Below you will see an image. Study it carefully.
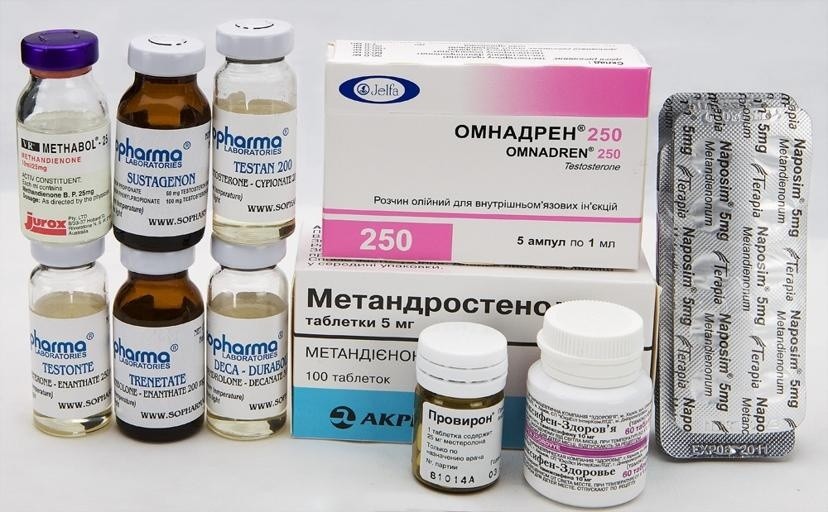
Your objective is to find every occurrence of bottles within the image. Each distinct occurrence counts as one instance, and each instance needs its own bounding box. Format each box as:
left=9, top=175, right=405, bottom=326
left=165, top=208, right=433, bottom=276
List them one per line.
left=205, top=233, right=288, bottom=441
left=113, top=243, right=205, bottom=443
left=16, top=28, right=111, bottom=245
left=522, top=298, right=653, bottom=508
left=212, top=18, right=299, bottom=246
left=28, top=237, right=112, bottom=438
left=410, top=321, right=509, bottom=494
left=112, top=31, right=211, bottom=253
